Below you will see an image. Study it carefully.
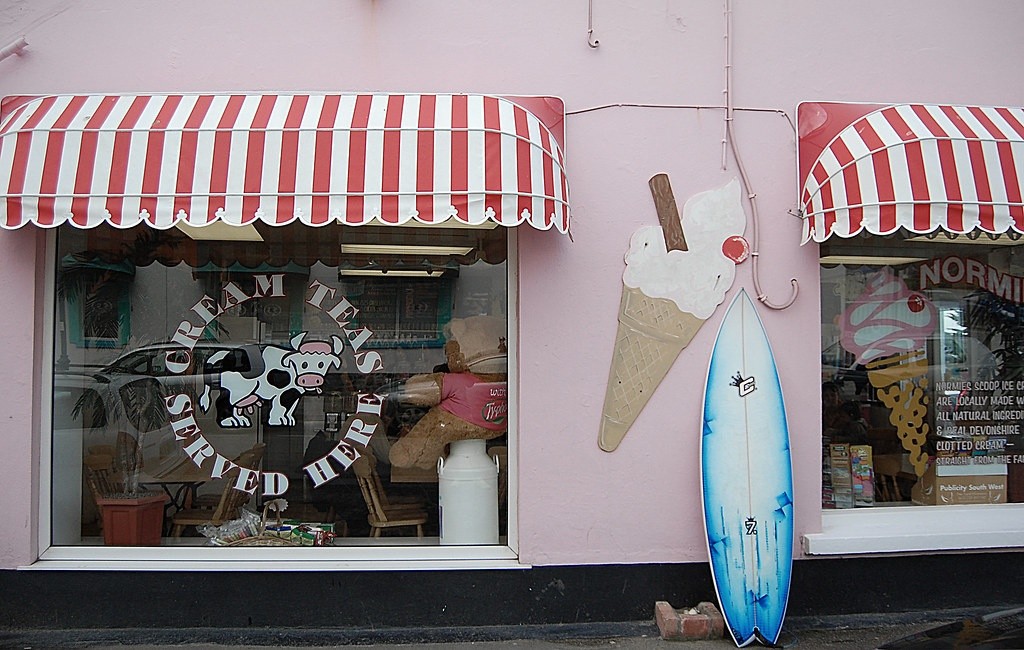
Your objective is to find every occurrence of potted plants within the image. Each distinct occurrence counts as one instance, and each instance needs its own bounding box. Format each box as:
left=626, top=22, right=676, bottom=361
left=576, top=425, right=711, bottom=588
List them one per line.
left=57, top=219, right=226, bottom=557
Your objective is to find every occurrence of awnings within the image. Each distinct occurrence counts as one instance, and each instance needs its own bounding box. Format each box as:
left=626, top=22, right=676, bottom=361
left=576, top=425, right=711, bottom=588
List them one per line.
left=0, top=93, right=574, bottom=238
left=794, top=101, right=1024, bottom=246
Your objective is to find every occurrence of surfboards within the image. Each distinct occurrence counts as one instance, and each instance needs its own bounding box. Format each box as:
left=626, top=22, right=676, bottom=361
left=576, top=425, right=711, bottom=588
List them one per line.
left=698, top=288, right=796, bottom=649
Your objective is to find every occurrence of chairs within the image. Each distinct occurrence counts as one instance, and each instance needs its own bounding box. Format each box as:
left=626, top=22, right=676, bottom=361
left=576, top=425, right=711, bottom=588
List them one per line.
left=85, top=452, right=132, bottom=528
left=348, top=445, right=432, bottom=539
left=169, top=443, right=267, bottom=537
left=868, top=451, right=903, bottom=503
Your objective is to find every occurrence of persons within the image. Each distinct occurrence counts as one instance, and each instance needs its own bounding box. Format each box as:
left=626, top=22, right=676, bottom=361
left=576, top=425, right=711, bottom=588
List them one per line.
left=822, top=362, right=969, bottom=460
left=305, top=393, right=416, bottom=540
left=160, top=345, right=249, bottom=372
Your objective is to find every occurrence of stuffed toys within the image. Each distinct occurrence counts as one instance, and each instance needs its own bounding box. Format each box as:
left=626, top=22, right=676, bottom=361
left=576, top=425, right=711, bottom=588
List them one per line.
left=390, top=314, right=508, bottom=470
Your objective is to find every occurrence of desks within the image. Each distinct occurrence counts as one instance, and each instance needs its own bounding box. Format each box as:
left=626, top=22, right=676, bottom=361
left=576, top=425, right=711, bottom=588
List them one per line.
left=388, top=459, right=439, bottom=492
left=126, top=448, right=234, bottom=539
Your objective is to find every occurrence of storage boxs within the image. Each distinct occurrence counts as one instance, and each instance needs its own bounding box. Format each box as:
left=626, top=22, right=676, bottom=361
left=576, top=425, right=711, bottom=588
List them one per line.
left=908, top=458, right=1009, bottom=505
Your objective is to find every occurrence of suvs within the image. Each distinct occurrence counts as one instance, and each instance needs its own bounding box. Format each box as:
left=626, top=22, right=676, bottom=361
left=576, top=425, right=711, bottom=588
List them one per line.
left=50, top=340, right=291, bottom=533
